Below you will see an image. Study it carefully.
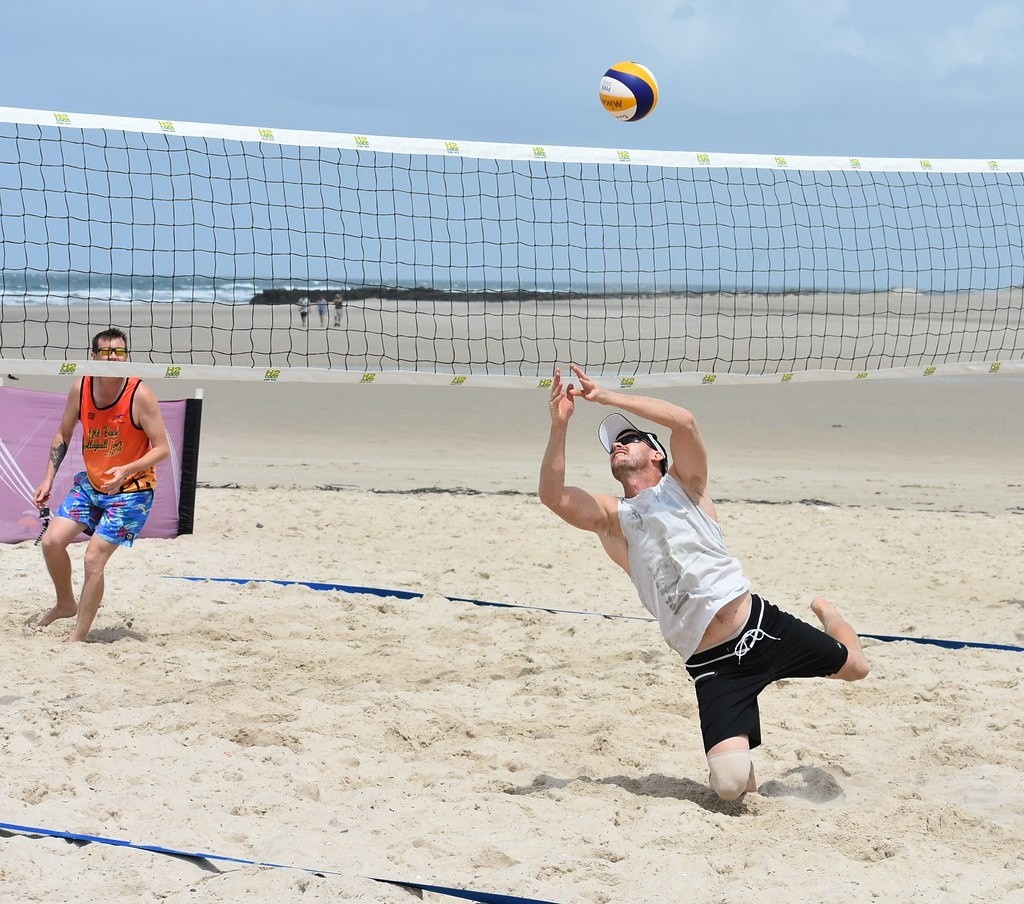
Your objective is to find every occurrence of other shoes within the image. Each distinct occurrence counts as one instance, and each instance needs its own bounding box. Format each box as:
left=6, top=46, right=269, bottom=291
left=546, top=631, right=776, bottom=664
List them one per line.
left=334, top=323, right=340, bottom=327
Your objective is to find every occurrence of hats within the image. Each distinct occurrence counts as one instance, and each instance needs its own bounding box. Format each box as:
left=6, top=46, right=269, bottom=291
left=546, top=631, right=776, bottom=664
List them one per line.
left=598, top=413, right=668, bottom=476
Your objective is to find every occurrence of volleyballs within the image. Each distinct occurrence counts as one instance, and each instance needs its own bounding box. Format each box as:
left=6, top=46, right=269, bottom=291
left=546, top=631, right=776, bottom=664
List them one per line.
left=598, top=60, right=659, bottom=123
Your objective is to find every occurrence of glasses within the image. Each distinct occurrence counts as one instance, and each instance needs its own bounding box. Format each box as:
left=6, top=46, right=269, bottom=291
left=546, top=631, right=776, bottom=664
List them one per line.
left=96, top=347, right=127, bottom=356
left=610, top=434, right=657, bottom=453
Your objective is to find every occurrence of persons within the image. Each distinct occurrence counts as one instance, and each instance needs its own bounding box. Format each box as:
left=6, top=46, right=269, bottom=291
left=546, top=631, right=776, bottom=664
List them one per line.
left=35, top=326, right=170, bottom=646
left=298, top=293, right=343, bottom=326
left=536, top=359, right=870, bottom=802
